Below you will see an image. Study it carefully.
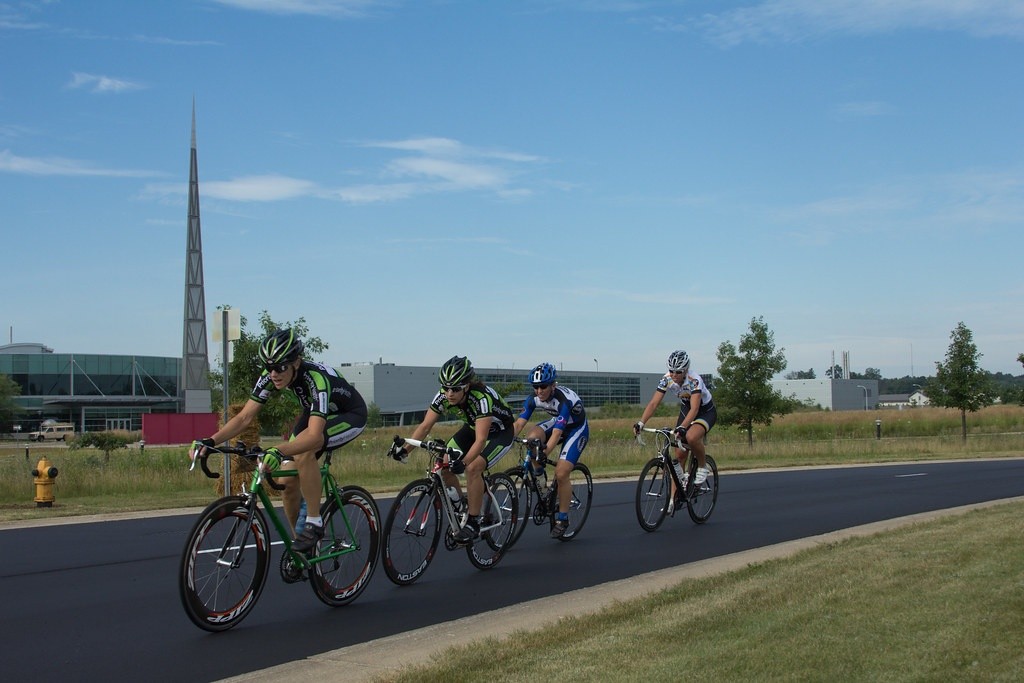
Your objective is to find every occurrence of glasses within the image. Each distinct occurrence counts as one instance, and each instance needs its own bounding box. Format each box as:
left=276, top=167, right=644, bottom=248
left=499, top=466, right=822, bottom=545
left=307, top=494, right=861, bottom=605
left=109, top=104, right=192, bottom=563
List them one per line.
left=532, top=383, right=552, bottom=389
left=441, top=385, right=467, bottom=392
left=265, top=362, right=292, bottom=373
left=669, top=369, right=685, bottom=374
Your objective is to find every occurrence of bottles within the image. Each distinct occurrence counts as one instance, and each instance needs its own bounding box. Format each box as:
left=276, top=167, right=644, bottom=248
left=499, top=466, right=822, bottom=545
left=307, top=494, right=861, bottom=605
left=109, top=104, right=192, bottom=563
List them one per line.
left=294, top=498, right=307, bottom=532
left=533, top=462, right=548, bottom=494
left=447, top=485, right=464, bottom=512
left=671, top=458, right=687, bottom=485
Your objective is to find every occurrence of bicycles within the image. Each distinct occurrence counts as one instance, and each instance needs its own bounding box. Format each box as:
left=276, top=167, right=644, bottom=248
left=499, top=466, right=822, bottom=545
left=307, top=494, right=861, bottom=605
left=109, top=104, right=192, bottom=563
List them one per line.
left=380, top=434, right=593, bottom=586
left=635, top=427, right=720, bottom=532
left=177, top=439, right=383, bottom=633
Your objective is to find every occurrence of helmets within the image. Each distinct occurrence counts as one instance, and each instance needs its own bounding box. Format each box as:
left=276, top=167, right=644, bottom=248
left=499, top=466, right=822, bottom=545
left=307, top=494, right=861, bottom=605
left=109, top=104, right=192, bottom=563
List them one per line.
left=667, top=350, right=691, bottom=372
left=437, top=355, right=476, bottom=386
left=258, top=328, right=304, bottom=365
left=527, top=362, right=557, bottom=385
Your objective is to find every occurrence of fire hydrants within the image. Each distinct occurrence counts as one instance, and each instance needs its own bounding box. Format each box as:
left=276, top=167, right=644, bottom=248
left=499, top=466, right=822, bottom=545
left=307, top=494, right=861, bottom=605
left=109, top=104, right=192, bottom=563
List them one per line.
left=31, top=456, right=58, bottom=508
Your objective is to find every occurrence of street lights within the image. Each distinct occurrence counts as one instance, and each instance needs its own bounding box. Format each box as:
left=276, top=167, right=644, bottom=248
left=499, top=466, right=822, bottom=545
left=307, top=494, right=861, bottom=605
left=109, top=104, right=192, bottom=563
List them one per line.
left=594, top=359, right=598, bottom=371
left=857, top=385, right=868, bottom=411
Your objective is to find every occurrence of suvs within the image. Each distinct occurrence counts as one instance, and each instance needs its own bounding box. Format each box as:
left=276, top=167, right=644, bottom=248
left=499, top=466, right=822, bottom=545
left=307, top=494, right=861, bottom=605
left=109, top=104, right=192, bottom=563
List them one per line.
left=28, top=426, right=75, bottom=442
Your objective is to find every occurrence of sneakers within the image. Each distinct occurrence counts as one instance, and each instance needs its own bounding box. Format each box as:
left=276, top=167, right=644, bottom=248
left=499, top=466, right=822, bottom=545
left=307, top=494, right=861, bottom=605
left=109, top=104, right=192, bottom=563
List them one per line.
left=291, top=523, right=324, bottom=551
left=531, top=471, right=547, bottom=493
left=453, top=523, right=480, bottom=543
left=550, top=517, right=570, bottom=539
left=661, top=500, right=673, bottom=513
left=694, top=467, right=710, bottom=484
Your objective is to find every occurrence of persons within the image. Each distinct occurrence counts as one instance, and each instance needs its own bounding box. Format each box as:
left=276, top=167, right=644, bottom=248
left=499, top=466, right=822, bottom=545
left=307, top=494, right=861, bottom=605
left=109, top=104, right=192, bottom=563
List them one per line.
left=187, top=328, right=368, bottom=570
left=633, top=350, right=717, bottom=514
left=392, top=355, right=515, bottom=543
left=514, top=362, right=589, bottom=539
